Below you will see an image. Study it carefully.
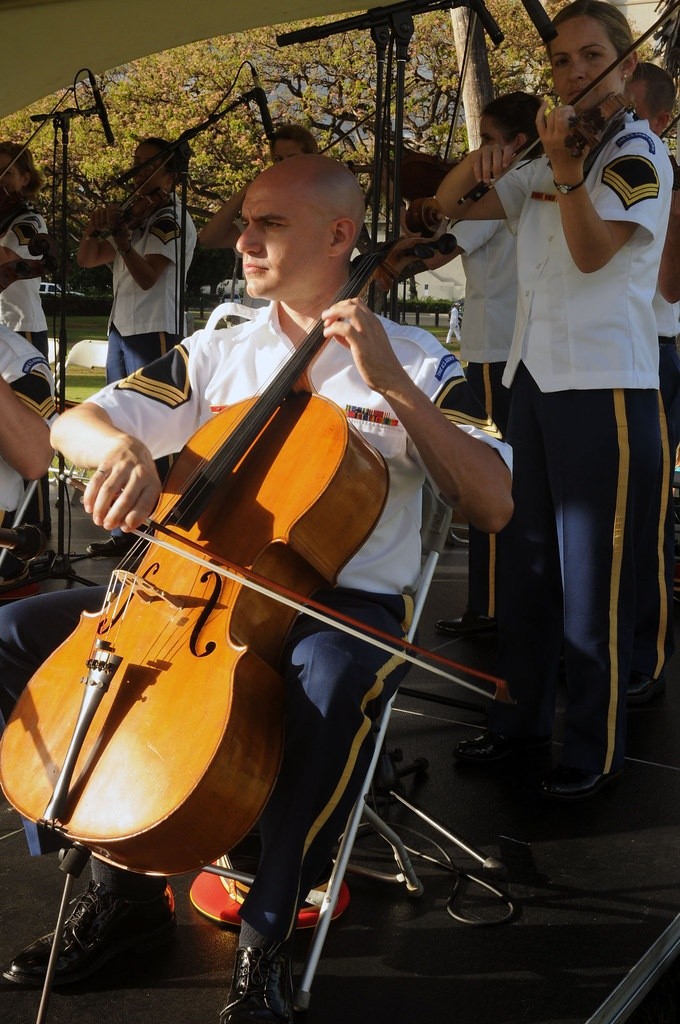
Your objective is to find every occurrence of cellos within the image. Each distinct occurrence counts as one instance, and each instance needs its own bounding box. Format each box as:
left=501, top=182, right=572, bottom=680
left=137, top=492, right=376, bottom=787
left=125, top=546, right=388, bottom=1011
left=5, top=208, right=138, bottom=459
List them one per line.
left=0, top=197, right=457, bottom=1024
left=0, top=233, right=60, bottom=294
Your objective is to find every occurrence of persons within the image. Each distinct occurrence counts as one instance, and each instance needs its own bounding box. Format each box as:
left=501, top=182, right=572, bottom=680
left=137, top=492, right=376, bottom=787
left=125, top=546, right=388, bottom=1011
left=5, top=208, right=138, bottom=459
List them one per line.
left=0, top=141, right=51, bottom=538
left=196, top=126, right=318, bottom=323
left=0, top=153, right=512, bottom=1024
left=380, top=0, right=680, bottom=793
left=0, top=324, right=59, bottom=556
left=446, top=303, right=463, bottom=344
left=77, top=137, right=196, bottom=556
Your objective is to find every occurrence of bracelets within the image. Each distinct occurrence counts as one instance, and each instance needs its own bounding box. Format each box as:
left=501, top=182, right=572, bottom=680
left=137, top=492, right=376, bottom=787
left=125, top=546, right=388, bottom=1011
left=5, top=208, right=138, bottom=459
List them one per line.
left=117, top=243, right=131, bottom=255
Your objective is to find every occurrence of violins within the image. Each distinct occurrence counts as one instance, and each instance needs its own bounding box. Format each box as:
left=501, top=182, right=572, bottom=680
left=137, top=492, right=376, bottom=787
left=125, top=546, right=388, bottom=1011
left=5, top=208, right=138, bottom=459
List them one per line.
left=126, top=184, right=171, bottom=230
left=546, top=91, right=630, bottom=171
left=0, top=191, right=27, bottom=234
left=348, top=152, right=459, bottom=200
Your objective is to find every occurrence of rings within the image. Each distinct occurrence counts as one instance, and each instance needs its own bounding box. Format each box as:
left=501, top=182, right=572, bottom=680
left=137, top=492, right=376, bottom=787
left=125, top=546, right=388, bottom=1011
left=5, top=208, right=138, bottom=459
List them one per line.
left=97, top=469, right=108, bottom=478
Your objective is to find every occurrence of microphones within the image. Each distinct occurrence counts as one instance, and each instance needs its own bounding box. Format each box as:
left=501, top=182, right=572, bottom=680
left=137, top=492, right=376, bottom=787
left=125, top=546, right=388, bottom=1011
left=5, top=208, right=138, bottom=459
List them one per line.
left=466, top=0, right=505, bottom=45
left=521, top=0, right=559, bottom=43
left=251, top=66, right=276, bottom=140
left=88, top=71, right=115, bottom=143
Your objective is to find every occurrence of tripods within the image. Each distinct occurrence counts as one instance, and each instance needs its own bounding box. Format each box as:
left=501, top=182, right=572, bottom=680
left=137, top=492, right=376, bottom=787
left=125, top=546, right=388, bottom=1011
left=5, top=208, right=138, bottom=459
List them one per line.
left=0, top=104, right=106, bottom=590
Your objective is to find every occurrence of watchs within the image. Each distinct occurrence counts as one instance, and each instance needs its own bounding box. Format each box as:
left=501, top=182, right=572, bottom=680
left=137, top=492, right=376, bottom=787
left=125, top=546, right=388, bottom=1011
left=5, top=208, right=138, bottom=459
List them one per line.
left=552, top=177, right=586, bottom=193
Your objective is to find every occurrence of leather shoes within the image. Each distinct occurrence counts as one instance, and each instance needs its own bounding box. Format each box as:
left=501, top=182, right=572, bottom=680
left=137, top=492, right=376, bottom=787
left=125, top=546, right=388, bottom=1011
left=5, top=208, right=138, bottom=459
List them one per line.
left=90, top=532, right=149, bottom=559
left=435, top=608, right=500, bottom=636
left=538, top=759, right=625, bottom=799
left=624, top=669, right=665, bottom=703
left=216, top=945, right=293, bottom=1024
left=452, top=720, right=552, bottom=761
left=4, top=878, right=176, bottom=986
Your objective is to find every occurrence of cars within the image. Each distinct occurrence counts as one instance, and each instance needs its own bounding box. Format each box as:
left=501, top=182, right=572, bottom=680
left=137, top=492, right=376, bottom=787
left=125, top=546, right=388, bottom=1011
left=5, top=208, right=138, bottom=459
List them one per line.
left=38, top=283, right=84, bottom=297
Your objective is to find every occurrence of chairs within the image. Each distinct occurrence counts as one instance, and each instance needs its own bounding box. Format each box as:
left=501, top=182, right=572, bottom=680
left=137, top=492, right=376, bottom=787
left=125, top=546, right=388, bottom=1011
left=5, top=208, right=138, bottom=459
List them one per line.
left=201, top=476, right=452, bottom=1011
left=0, top=338, right=109, bottom=565
